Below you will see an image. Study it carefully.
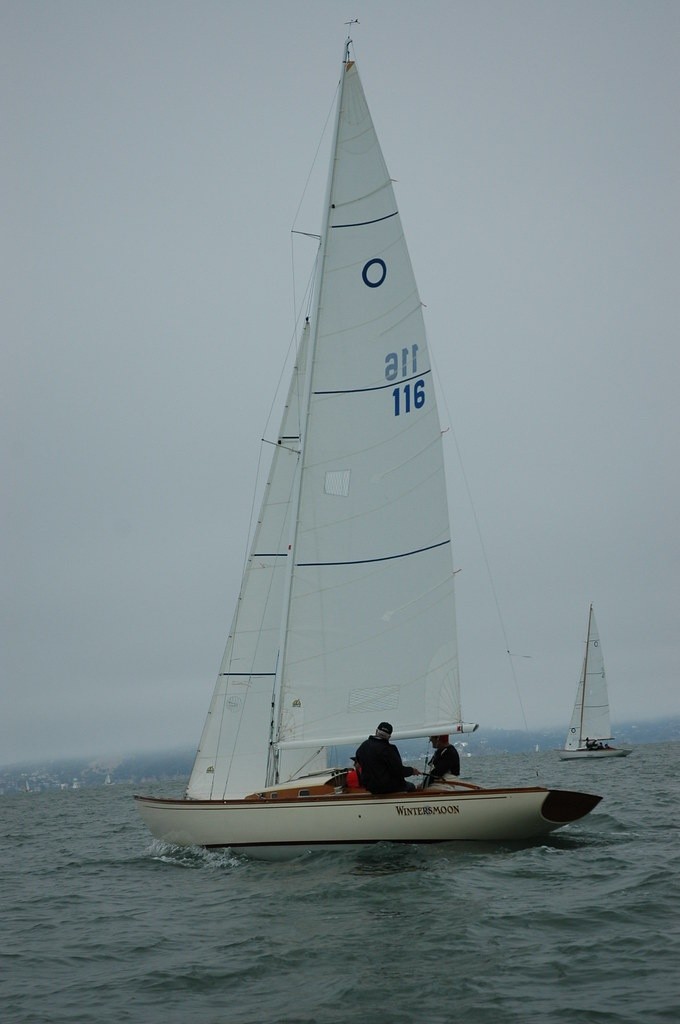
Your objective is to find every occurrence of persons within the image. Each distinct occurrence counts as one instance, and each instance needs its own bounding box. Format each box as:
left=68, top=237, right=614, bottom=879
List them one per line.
left=343, top=756, right=367, bottom=794
left=586, top=737, right=611, bottom=750
left=356, top=721, right=419, bottom=796
left=417, top=734, right=460, bottom=791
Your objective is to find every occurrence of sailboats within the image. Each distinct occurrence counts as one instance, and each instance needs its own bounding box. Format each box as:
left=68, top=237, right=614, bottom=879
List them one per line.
left=129, top=18, right=606, bottom=862
left=553, top=600, right=633, bottom=761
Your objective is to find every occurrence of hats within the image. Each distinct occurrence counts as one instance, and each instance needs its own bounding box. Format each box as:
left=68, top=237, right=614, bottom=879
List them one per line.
left=378, top=722, right=393, bottom=734
